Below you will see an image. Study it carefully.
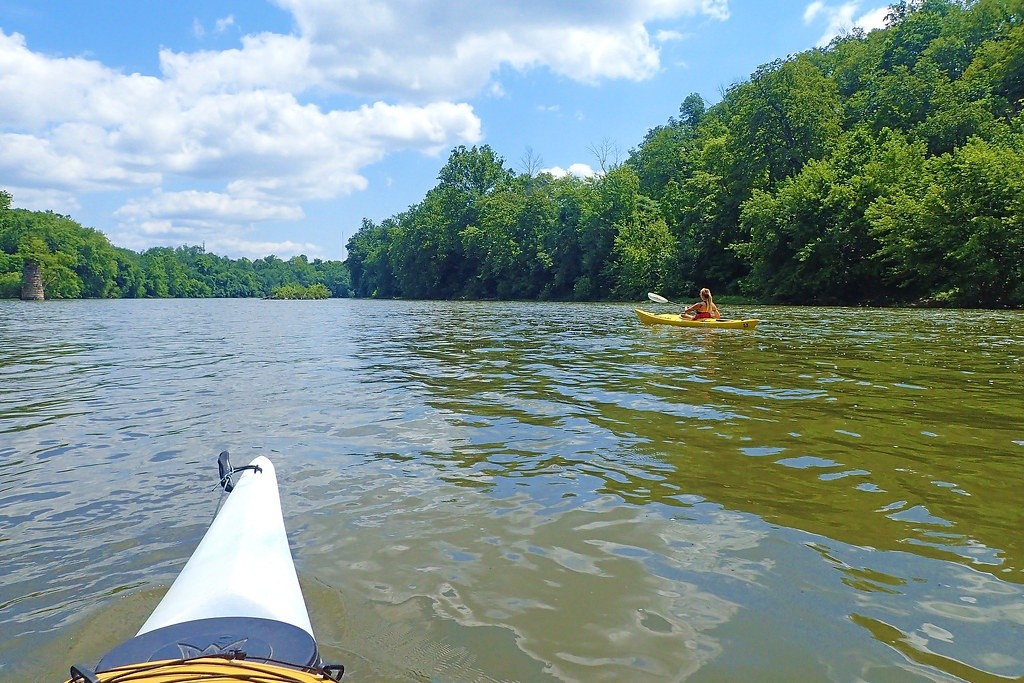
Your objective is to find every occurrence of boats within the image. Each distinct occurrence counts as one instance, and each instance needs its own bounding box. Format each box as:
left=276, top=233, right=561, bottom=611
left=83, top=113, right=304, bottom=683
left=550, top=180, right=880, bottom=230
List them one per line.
left=632, top=305, right=760, bottom=330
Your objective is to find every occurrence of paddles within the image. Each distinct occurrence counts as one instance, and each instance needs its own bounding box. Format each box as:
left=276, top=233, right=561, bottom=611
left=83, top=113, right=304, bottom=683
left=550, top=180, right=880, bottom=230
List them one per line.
left=648, top=292, right=688, bottom=309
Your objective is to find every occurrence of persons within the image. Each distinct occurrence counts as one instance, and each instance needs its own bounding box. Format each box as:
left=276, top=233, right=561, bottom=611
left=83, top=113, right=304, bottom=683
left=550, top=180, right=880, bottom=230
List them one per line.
left=681, top=288, right=720, bottom=320
